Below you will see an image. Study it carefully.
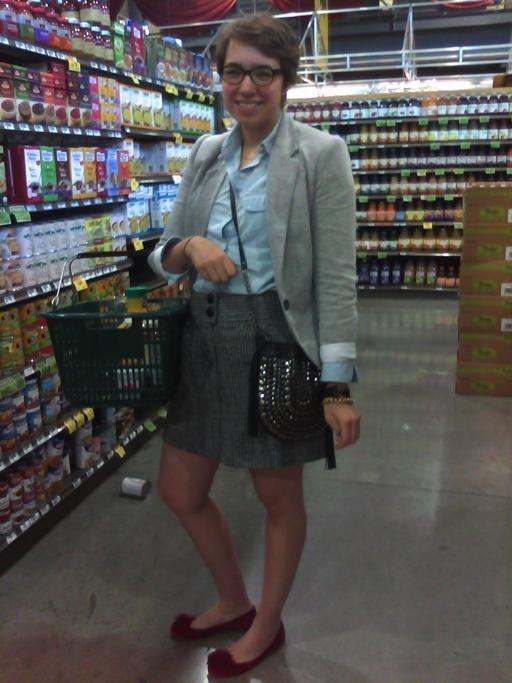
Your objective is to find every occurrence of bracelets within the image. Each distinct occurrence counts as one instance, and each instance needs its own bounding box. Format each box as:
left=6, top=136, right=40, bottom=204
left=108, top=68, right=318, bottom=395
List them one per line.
left=183, top=233, right=199, bottom=260
left=321, top=394, right=354, bottom=405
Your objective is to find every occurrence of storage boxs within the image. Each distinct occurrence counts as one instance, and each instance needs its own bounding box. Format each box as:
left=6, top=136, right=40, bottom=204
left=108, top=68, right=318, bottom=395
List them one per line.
left=453, top=186, right=511, bottom=399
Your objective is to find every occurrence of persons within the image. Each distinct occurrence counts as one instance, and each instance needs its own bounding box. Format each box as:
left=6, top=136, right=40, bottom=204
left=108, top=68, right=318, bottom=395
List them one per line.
left=147, top=11, right=359, bottom=677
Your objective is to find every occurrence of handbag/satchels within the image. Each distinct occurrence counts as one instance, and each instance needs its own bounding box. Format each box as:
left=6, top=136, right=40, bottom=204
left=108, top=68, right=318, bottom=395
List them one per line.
left=256, top=340, right=328, bottom=444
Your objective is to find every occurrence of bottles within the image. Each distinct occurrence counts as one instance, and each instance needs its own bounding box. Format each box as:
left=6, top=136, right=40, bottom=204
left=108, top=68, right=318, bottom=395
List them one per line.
left=113, top=284, right=158, bottom=366
left=288, top=91, right=511, bottom=294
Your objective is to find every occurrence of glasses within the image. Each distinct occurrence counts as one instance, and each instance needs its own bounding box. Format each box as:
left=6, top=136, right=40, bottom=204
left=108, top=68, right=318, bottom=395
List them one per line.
left=218, top=64, right=280, bottom=86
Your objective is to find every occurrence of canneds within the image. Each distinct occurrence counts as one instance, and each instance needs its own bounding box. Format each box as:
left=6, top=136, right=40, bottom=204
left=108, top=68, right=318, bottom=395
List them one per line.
left=0, top=212, right=116, bottom=538
left=0, top=0, right=116, bottom=66
left=121, top=476, right=148, bottom=499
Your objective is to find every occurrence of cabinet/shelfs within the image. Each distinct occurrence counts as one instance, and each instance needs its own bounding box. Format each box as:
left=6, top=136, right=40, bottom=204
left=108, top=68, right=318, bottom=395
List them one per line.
left=1, top=36, right=237, bottom=579
left=286, top=84, right=511, bottom=295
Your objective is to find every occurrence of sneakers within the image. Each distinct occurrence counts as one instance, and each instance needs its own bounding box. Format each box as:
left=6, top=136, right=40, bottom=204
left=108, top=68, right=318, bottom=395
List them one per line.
left=170, top=606, right=257, bottom=640
left=208, top=621, right=285, bottom=676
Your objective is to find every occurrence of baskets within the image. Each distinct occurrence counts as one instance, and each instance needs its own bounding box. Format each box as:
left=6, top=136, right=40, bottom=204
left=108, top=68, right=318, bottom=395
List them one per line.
left=47, top=249, right=189, bottom=409
